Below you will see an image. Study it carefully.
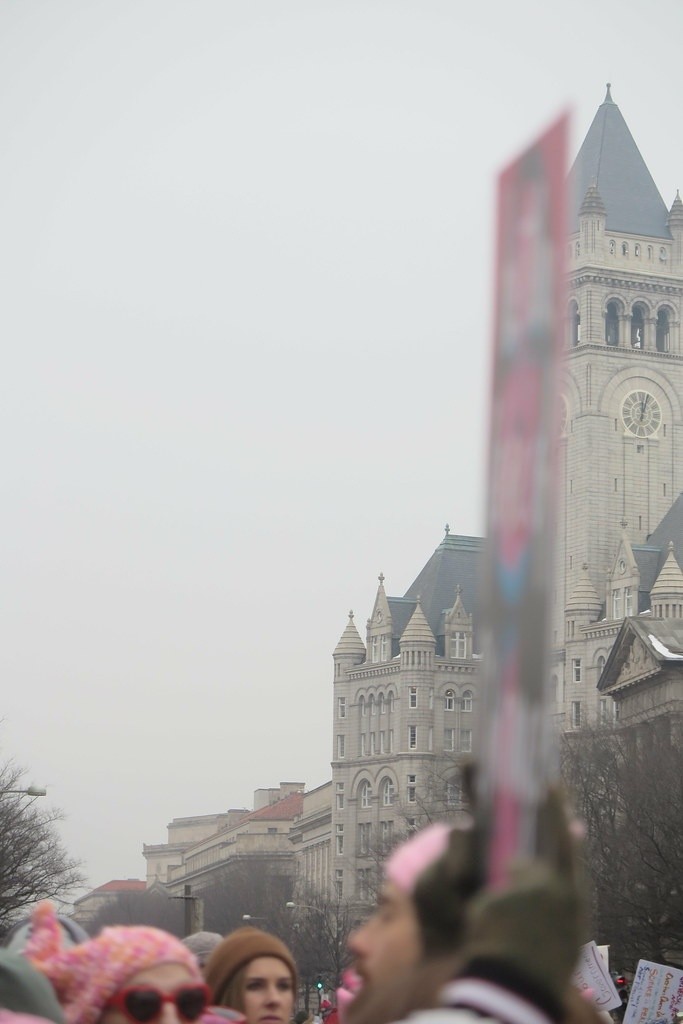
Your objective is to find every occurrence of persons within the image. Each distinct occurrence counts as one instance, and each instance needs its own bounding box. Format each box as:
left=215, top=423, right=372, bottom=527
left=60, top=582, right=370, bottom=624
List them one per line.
left=182, top=932, right=225, bottom=972
left=205, top=927, right=298, bottom=1024
left=0, top=915, right=208, bottom=1024
left=614, top=976, right=629, bottom=1021
left=295, top=759, right=606, bottom=1023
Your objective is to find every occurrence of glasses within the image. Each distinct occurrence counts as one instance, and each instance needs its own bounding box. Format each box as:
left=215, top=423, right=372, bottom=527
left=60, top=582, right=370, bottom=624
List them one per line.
left=104, top=983, right=209, bottom=1024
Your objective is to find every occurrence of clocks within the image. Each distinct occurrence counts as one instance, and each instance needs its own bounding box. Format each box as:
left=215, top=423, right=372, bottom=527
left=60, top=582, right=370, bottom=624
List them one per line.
left=619, top=389, right=662, bottom=438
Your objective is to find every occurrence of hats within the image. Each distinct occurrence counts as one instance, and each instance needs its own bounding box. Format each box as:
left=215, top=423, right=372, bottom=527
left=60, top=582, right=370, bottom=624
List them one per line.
left=26, top=901, right=202, bottom=1024
left=204, top=926, right=297, bottom=994
left=379, top=791, right=592, bottom=981
left=181, top=930, right=225, bottom=964
